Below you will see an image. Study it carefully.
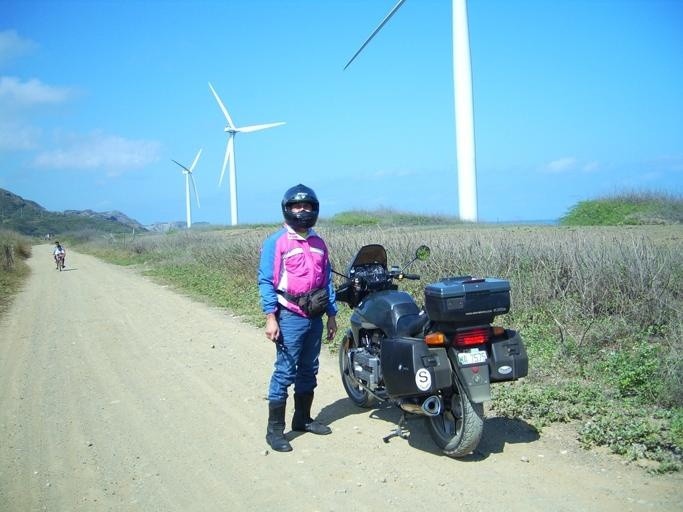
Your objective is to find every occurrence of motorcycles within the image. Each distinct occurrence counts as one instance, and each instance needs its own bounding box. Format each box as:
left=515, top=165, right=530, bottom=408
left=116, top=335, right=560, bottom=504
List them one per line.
left=326, top=242, right=531, bottom=461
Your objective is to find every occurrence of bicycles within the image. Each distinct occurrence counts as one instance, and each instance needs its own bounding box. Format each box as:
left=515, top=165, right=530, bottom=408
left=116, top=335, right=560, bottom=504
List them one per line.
left=54, top=253, right=66, bottom=271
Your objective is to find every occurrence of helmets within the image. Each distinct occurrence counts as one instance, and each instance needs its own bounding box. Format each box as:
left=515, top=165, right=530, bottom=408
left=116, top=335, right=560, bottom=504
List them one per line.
left=280, top=184, right=320, bottom=229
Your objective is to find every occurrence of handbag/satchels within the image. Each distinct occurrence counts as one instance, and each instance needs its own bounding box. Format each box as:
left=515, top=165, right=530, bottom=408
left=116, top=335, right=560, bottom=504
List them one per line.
left=274, top=287, right=331, bottom=318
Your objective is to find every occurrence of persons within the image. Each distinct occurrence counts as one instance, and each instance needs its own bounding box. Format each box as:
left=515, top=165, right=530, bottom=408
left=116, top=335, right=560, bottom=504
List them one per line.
left=52, top=241, right=65, bottom=269
left=257, top=184, right=338, bottom=453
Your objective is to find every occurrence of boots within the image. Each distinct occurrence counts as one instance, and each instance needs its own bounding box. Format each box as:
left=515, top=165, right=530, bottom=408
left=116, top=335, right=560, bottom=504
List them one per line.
left=291, top=392, right=333, bottom=436
left=266, top=399, right=293, bottom=452
